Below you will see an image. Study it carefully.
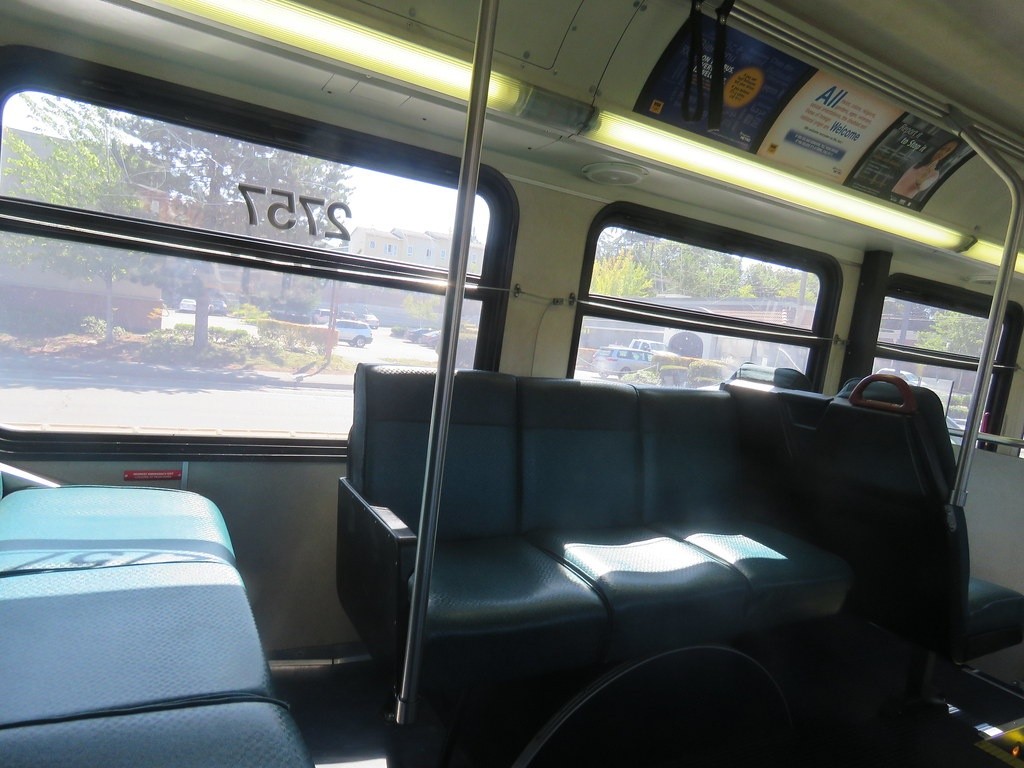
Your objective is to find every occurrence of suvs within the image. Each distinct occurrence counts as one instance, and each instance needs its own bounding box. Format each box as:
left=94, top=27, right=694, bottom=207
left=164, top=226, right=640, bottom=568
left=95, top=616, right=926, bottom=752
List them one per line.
left=325, top=319, right=374, bottom=348
left=592, top=347, right=657, bottom=381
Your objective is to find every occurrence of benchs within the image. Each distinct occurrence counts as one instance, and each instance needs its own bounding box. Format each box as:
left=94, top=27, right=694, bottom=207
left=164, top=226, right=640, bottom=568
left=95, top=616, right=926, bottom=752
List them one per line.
left=0, top=473, right=315, bottom=768
left=720, top=366, right=1024, bottom=718
left=334, top=363, right=855, bottom=768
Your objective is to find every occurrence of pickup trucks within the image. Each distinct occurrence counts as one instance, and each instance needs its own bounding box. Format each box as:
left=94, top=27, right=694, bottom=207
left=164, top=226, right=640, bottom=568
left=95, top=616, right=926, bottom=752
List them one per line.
left=609, top=339, right=666, bottom=354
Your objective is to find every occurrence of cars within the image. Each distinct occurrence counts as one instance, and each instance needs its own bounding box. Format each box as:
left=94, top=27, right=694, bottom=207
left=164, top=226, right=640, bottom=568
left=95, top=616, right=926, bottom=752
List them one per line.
left=208, top=300, right=229, bottom=318
left=359, top=313, right=380, bottom=329
left=406, top=327, right=442, bottom=348
left=180, top=299, right=197, bottom=313
left=311, top=308, right=334, bottom=325
